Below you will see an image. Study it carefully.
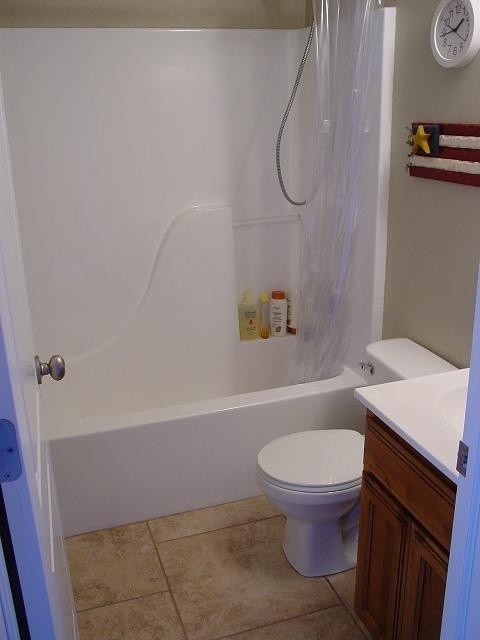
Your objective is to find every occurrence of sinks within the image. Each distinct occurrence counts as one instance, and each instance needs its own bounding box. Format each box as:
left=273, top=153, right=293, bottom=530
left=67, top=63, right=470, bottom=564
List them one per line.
left=441, top=384, right=468, bottom=431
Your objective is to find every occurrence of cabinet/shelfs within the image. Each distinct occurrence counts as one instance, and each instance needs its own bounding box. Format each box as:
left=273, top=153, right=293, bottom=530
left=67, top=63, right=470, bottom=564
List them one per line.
left=353, top=410, right=458, bottom=640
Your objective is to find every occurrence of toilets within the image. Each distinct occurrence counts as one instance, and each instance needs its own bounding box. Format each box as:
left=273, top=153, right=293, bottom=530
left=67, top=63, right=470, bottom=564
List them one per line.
left=254, top=335, right=460, bottom=576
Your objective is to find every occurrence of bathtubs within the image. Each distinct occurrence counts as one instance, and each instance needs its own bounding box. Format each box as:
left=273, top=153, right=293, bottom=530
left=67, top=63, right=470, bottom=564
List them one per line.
left=45, top=347, right=368, bottom=540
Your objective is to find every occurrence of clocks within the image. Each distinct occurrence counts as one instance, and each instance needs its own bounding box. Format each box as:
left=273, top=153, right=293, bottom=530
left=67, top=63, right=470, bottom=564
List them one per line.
left=428, top=2, right=479, bottom=71
